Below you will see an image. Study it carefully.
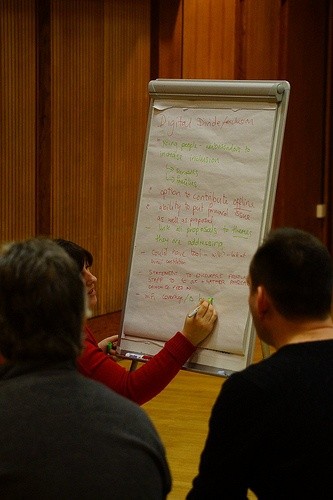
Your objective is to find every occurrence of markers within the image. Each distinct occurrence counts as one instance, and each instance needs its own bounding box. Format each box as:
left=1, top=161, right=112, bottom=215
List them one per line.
left=188, top=297, right=214, bottom=318
left=125, top=353, right=153, bottom=361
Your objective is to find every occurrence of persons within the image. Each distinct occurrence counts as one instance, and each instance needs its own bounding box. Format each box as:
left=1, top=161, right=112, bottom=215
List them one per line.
left=183, top=228, right=333, bottom=500
left=52, top=240, right=218, bottom=406
left=0, top=238, right=173, bottom=500
